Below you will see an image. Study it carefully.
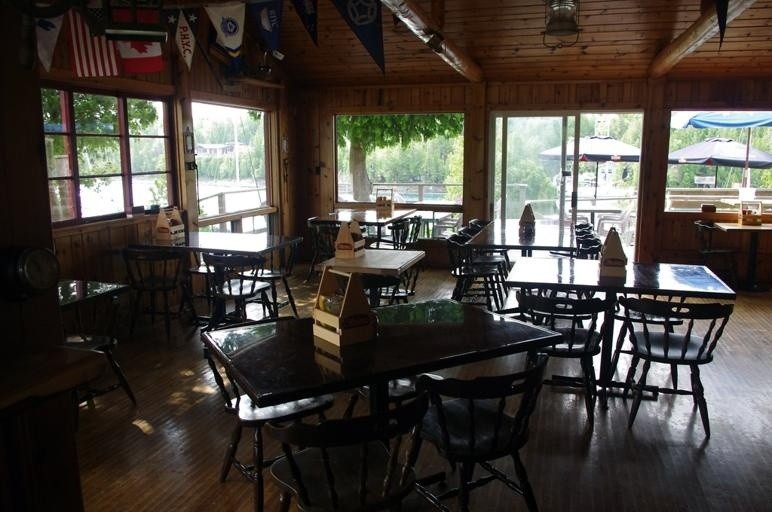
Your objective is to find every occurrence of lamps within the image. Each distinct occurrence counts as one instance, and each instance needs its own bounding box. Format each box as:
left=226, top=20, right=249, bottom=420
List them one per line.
left=539, top=0, right=586, bottom=49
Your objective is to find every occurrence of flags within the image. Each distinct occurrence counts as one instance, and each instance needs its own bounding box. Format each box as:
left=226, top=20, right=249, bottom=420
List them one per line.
left=64, top=0, right=120, bottom=78
left=204, top=1, right=246, bottom=77
left=331, top=0, right=385, bottom=76
left=715, top=0, right=730, bottom=51
left=32, top=0, right=66, bottom=72
left=289, top=1, right=319, bottom=48
left=164, top=4, right=202, bottom=73
left=245, top=0, right=281, bottom=50
left=107, top=0, right=167, bottom=73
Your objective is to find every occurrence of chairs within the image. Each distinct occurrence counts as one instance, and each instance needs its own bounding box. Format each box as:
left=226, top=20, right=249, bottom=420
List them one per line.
left=305, top=219, right=345, bottom=280
left=412, top=350, right=551, bottom=512
left=515, top=290, right=619, bottom=424
left=616, top=294, right=735, bottom=440
left=201, top=252, right=274, bottom=332
left=341, top=371, right=448, bottom=422
left=445, top=217, right=513, bottom=310
left=388, top=216, right=423, bottom=251
left=202, top=314, right=337, bottom=512
left=121, top=245, right=200, bottom=335
left=70, top=271, right=137, bottom=430
left=377, top=262, right=421, bottom=305
left=692, top=219, right=741, bottom=287
left=262, top=385, right=430, bottom=512
left=262, top=234, right=305, bottom=319
left=575, top=222, right=602, bottom=261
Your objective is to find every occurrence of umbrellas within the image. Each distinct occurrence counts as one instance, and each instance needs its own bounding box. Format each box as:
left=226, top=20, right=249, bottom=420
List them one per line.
left=671, top=112, right=772, bottom=188
left=539, top=135, right=640, bottom=232
left=668, top=138, right=772, bottom=188
